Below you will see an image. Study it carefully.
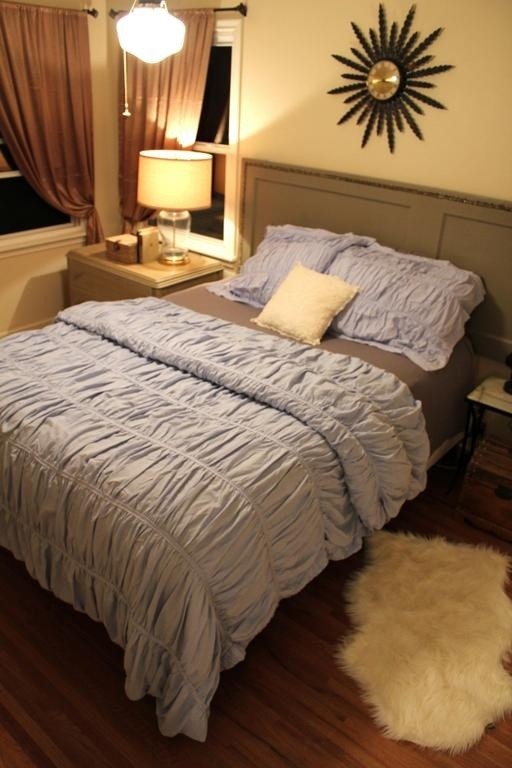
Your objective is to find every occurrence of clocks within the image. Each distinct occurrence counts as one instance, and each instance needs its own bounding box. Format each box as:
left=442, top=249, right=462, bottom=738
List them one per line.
left=327, top=3, right=456, bottom=154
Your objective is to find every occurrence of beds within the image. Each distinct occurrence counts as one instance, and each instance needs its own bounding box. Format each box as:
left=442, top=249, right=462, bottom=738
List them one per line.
left=0, top=158, right=512, bottom=742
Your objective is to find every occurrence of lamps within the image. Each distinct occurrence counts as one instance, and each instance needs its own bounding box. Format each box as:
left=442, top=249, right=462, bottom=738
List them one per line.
left=137, top=149, right=212, bottom=265
left=116, top=0, right=186, bottom=65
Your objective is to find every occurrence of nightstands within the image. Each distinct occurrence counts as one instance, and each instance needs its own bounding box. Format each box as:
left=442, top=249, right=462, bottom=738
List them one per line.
left=66, top=243, right=225, bottom=306
left=459, top=376, right=512, bottom=543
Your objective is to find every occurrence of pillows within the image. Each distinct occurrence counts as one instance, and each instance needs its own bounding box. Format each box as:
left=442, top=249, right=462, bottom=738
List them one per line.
left=249, top=262, right=360, bottom=346
left=322, top=242, right=486, bottom=372
left=205, top=223, right=377, bottom=309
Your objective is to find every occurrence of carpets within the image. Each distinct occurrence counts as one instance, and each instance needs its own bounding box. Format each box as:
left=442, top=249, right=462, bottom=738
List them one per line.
left=333, top=526, right=512, bottom=756
